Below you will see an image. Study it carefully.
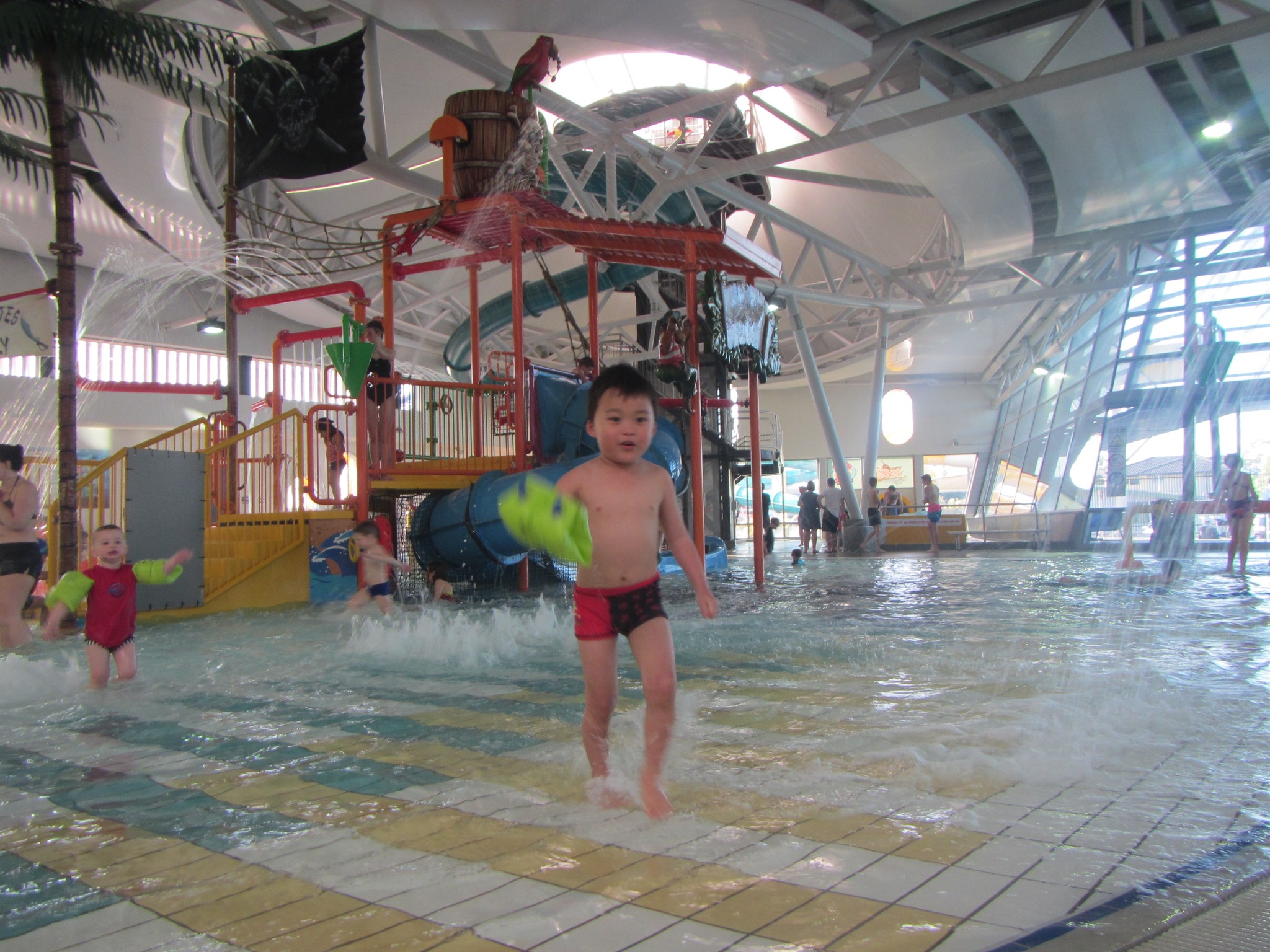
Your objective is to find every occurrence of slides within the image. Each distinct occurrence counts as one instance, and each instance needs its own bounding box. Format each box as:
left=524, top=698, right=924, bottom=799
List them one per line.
left=441, top=80, right=760, bottom=389
left=410, top=361, right=729, bottom=587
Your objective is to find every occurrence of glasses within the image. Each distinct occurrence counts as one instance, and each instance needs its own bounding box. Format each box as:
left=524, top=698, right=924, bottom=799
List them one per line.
left=791, top=553, right=795, bottom=556
left=774, top=523, right=780, bottom=526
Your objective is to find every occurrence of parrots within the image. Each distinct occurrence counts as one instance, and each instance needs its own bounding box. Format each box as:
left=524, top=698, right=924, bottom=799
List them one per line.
left=504, top=35, right=558, bottom=98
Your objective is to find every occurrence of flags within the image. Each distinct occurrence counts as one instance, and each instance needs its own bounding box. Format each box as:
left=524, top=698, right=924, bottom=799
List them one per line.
left=232, top=26, right=368, bottom=193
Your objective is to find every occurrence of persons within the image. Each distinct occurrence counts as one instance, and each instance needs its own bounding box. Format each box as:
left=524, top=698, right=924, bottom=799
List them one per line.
left=0, top=444, right=41, bottom=649
left=801, top=481, right=821, bottom=553
left=571, top=357, right=596, bottom=383
left=427, top=561, right=458, bottom=604
left=836, top=505, right=849, bottom=552
left=315, top=417, right=346, bottom=510
left=40, top=524, right=193, bottom=692
left=761, top=483, right=771, bottom=531
left=657, top=310, right=700, bottom=416
left=790, top=549, right=804, bottom=565
left=921, top=474, right=942, bottom=552
left=763, top=517, right=779, bottom=555
left=798, top=486, right=807, bottom=546
left=816, top=478, right=845, bottom=552
left=1059, top=559, right=1181, bottom=585
left=1216, top=453, right=1258, bottom=573
left=345, top=520, right=408, bottom=617
left=363, top=319, right=394, bottom=481
left=860, top=477, right=886, bottom=553
left=553, top=363, right=716, bottom=820
left=882, top=485, right=903, bottom=515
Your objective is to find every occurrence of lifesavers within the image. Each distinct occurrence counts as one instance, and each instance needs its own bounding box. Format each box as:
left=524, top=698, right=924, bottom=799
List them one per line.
left=131, top=557, right=184, bottom=585
left=496, top=471, right=598, bottom=570
left=43, top=569, right=96, bottom=614
